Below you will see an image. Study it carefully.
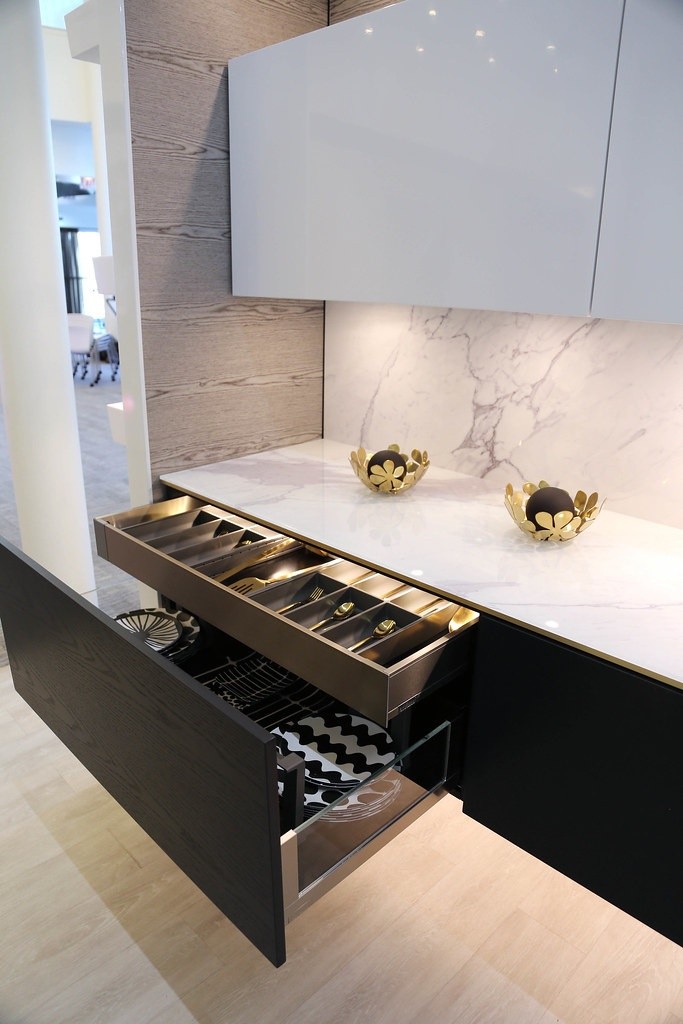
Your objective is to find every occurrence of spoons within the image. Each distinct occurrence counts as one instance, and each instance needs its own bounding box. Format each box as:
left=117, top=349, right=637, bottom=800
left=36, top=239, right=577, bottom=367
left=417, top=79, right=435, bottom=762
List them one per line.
left=347, top=619, right=396, bottom=651
left=308, top=602, right=354, bottom=631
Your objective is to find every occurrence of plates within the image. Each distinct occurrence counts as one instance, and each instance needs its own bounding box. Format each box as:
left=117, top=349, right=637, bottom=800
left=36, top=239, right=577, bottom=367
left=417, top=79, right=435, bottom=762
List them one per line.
left=113, top=607, right=201, bottom=665
left=271, top=714, right=403, bottom=822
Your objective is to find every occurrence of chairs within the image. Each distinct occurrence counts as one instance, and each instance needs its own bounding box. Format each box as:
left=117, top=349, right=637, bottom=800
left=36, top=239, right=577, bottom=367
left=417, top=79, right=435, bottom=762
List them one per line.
left=68, top=313, right=93, bottom=380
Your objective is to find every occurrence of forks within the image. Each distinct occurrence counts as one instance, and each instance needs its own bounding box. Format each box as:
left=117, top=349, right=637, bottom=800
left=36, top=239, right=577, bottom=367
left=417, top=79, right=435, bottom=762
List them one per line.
left=275, top=586, right=324, bottom=614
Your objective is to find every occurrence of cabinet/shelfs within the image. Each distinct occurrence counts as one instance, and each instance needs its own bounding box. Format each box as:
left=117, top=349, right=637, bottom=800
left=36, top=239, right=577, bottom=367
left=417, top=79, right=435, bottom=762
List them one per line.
left=0, top=0, right=683, bottom=968
